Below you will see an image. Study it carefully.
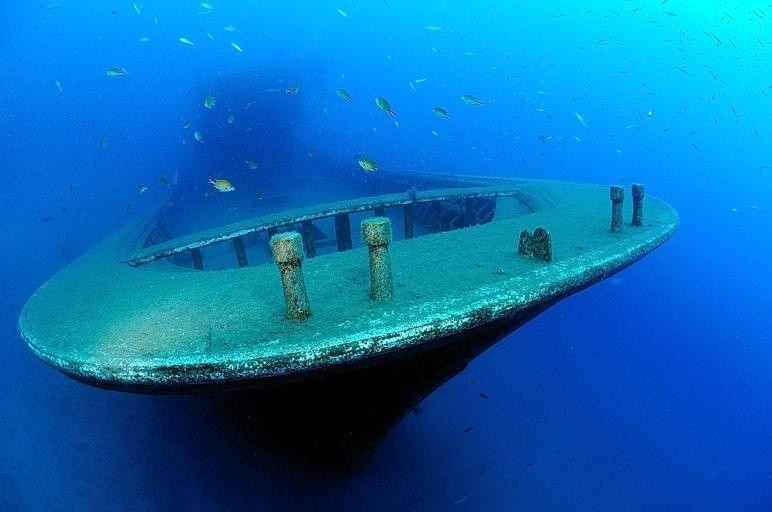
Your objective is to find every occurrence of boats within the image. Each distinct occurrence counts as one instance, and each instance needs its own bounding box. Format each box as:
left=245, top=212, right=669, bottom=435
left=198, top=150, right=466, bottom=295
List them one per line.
left=18, top=68, right=680, bottom=486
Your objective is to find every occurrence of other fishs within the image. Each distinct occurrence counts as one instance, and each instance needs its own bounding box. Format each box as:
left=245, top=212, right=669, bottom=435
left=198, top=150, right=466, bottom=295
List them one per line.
left=41, top=0, right=772, bottom=224
